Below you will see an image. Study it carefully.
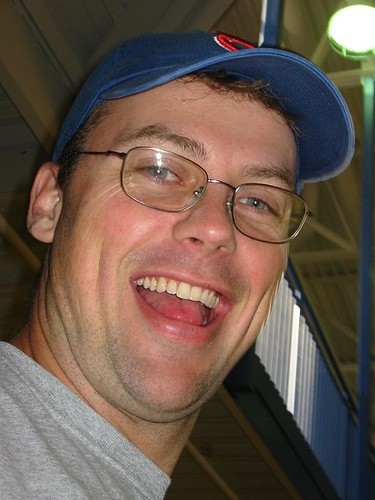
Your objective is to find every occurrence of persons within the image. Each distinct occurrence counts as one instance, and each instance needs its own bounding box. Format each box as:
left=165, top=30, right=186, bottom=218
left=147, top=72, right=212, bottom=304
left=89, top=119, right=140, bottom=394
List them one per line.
left=0, top=32, right=356, bottom=500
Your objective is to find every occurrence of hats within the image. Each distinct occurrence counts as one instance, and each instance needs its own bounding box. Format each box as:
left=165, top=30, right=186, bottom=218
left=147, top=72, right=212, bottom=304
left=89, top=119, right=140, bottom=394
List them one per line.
left=50, top=31, right=357, bottom=193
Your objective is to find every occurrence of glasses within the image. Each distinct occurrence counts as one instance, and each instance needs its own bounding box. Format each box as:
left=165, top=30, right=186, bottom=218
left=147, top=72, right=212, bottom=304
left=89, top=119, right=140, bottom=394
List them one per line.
left=75, top=147, right=314, bottom=243
